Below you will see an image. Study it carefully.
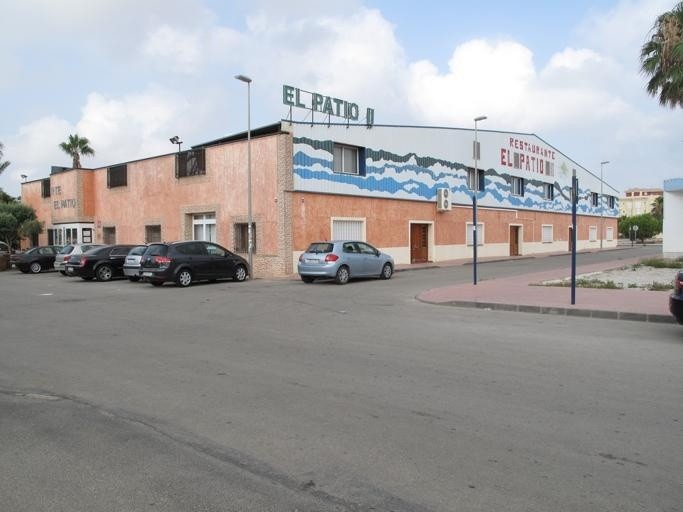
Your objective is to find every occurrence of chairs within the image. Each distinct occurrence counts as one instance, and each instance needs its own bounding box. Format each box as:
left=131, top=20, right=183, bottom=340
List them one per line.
left=326, top=243, right=355, bottom=252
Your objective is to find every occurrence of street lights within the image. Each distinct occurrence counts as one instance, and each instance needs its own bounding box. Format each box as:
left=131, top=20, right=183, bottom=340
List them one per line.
left=232, top=72, right=254, bottom=283
left=472, top=115, right=487, bottom=283
left=599, top=159, right=611, bottom=250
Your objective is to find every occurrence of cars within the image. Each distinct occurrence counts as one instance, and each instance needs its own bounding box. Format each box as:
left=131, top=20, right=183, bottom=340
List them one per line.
left=667, top=270, right=683, bottom=327
left=63, top=244, right=141, bottom=283
left=9, top=244, right=63, bottom=275
left=138, top=240, right=251, bottom=291
left=123, top=240, right=147, bottom=283
left=296, top=238, right=395, bottom=286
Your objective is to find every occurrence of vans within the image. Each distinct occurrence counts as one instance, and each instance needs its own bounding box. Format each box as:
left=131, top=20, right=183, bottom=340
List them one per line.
left=0, top=240, right=17, bottom=271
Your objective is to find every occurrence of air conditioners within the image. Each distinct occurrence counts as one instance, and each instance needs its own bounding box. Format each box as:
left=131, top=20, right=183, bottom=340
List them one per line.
left=436, top=187, right=452, bottom=212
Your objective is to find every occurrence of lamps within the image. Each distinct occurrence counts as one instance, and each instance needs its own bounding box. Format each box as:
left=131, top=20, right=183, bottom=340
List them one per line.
left=170, top=136, right=183, bottom=152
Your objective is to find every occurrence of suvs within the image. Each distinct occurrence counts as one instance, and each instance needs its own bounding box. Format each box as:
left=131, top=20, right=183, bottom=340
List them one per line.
left=53, top=242, right=113, bottom=277
